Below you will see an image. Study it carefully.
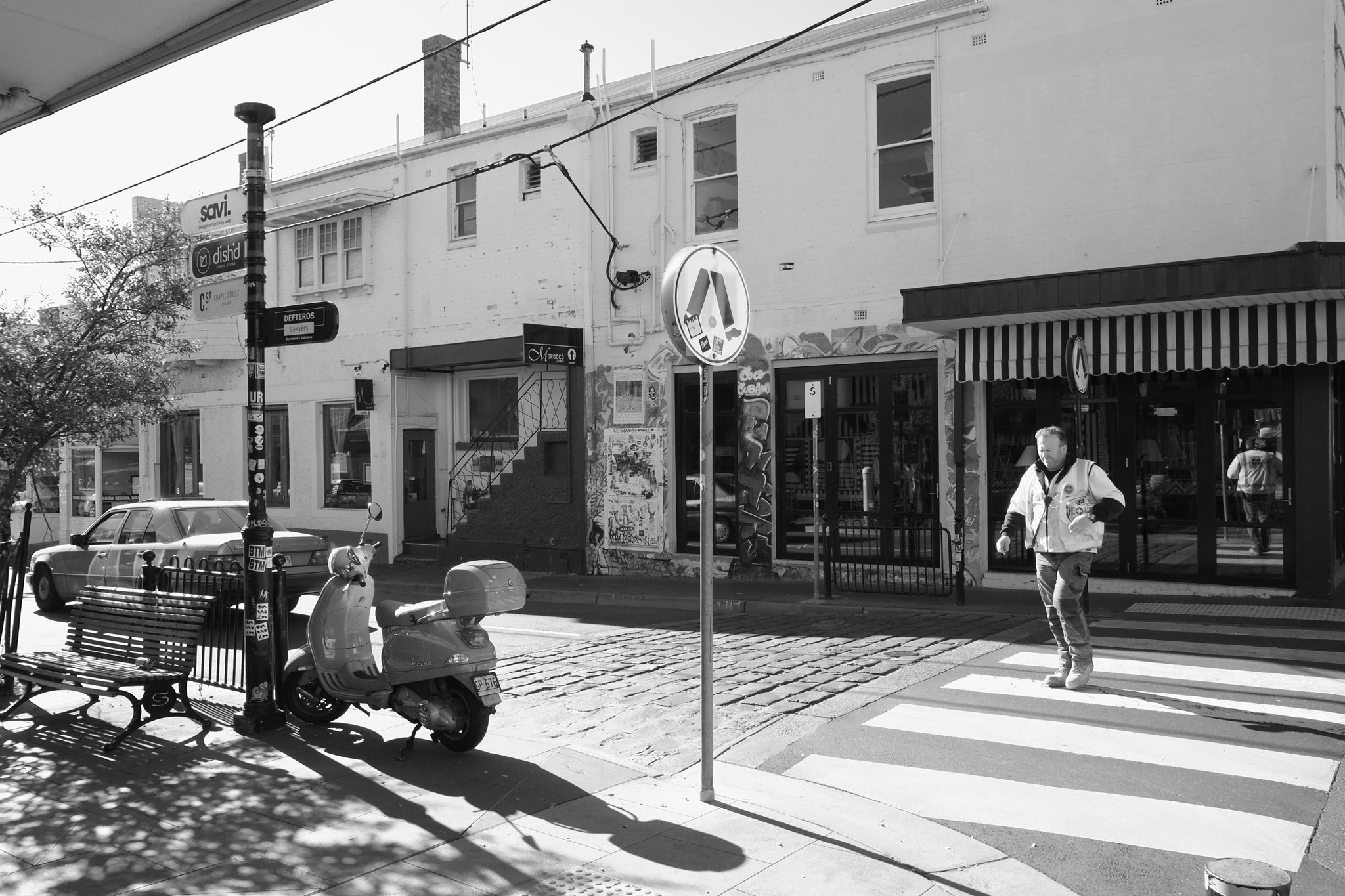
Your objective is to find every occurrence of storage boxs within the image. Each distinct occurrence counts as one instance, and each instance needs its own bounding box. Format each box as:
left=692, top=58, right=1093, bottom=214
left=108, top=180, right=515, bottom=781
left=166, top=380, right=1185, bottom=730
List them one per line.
left=443, top=558, right=528, bottom=615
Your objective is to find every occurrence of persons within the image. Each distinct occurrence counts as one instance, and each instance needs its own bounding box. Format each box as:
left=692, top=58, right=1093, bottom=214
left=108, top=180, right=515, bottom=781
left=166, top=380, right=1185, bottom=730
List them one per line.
left=995, top=424, right=1128, bottom=692
left=1227, top=436, right=1285, bottom=557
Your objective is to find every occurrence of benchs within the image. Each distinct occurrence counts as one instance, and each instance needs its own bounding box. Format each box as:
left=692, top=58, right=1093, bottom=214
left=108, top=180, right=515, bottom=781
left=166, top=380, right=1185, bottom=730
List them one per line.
left=0, top=583, right=221, bottom=751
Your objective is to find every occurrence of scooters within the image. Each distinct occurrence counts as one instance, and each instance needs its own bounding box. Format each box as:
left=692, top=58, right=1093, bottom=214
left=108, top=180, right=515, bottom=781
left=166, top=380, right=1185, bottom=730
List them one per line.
left=1136, top=474, right=1168, bottom=522
left=279, top=502, right=526, bottom=761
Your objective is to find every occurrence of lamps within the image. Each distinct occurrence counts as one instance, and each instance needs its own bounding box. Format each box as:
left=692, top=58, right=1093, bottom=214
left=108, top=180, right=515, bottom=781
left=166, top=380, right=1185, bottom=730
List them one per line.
left=378, top=363, right=388, bottom=374
left=352, top=364, right=362, bottom=377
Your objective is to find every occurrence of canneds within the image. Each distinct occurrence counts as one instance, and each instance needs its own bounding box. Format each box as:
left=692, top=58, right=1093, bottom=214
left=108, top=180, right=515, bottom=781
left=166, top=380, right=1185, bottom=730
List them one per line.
left=135, top=657, right=154, bottom=669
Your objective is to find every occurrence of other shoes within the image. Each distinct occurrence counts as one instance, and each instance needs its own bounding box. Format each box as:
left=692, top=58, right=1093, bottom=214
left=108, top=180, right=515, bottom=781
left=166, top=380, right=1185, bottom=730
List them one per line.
left=1065, top=661, right=1094, bottom=689
left=1044, top=666, right=1071, bottom=686
left=1249, top=548, right=1262, bottom=556
left=1262, top=546, right=1269, bottom=552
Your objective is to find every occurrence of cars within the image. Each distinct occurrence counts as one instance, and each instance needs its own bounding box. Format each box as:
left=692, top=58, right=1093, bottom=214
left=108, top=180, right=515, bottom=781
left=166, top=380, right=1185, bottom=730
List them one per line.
left=24, top=500, right=329, bottom=630
left=687, top=473, right=736, bottom=543
